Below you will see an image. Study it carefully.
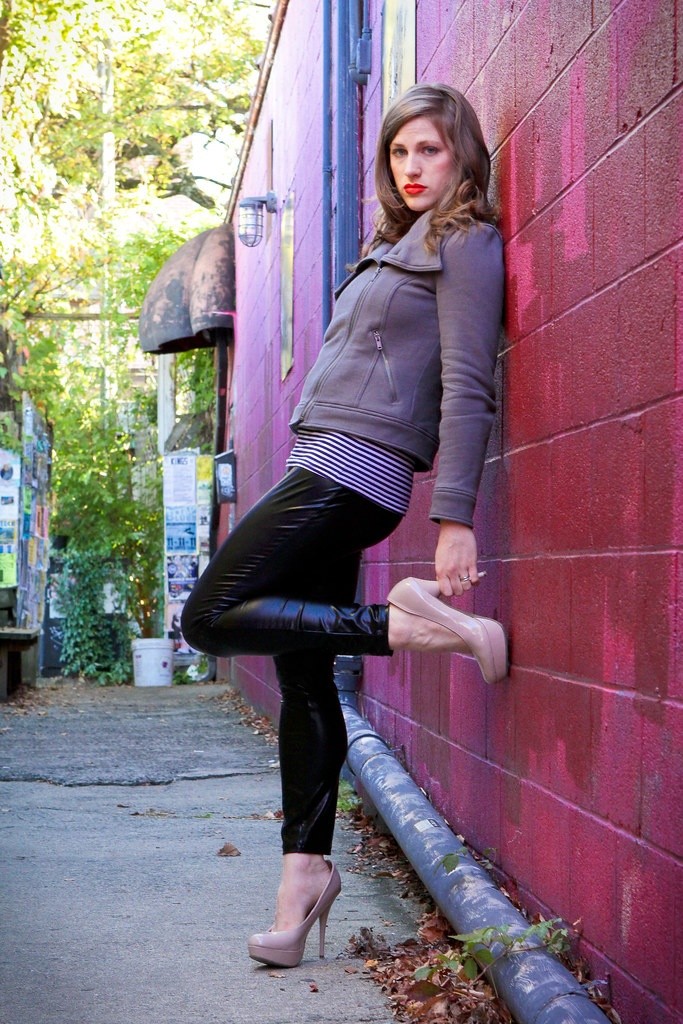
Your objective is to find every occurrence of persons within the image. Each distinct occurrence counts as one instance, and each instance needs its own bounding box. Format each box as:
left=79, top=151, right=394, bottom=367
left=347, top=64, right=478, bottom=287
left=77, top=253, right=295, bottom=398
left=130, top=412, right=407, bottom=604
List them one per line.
left=180, top=83, right=509, bottom=968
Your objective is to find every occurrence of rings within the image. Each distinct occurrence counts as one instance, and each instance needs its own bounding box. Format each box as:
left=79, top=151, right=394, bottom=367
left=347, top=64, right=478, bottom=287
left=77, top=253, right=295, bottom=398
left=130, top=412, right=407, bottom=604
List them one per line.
left=460, top=577, right=468, bottom=582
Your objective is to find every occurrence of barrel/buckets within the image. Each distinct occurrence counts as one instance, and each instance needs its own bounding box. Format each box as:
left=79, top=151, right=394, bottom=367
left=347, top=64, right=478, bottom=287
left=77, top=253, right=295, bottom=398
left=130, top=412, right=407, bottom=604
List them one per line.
left=131, top=638, right=175, bottom=687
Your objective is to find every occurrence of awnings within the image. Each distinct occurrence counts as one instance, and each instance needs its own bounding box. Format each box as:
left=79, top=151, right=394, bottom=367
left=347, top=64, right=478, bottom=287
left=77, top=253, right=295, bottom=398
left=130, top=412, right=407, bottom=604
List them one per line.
left=138, top=225, right=234, bottom=353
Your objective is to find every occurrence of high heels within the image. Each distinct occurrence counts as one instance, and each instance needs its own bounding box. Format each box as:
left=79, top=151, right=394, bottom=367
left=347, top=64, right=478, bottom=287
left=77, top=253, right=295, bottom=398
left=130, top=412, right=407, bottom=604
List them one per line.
left=248, top=859, right=341, bottom=966
left=386, top=570, right=507, bottom=683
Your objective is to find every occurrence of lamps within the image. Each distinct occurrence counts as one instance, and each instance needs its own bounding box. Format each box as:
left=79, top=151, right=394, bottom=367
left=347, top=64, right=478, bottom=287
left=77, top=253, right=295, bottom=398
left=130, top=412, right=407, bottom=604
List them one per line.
left=238, top=192, right=277, bottom=246
